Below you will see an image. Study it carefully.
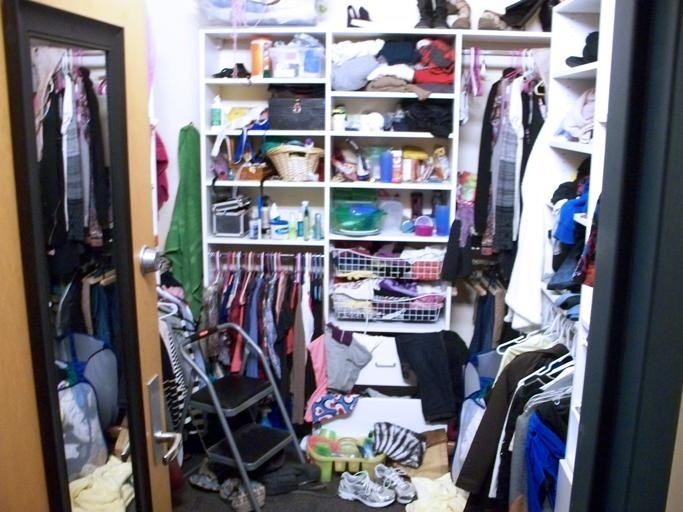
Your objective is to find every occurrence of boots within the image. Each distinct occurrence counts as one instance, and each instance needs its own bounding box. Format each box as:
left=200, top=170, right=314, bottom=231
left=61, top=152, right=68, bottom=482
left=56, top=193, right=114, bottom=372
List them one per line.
left=431, top=0, right=450, bottom=29
left=414, top=0, right=432, bottom=28
left=506, top=0, right=559, bottom=31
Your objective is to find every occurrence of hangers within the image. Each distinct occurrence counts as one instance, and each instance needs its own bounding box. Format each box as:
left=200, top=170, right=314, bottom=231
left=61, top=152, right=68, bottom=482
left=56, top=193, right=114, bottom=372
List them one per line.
left=495, top=306, right=577, bottom=419
left=462, top=43, right=485, bottom=99
left=492, top=44, right=546, bottom=95
left=205, top=245, right=323, bottom=314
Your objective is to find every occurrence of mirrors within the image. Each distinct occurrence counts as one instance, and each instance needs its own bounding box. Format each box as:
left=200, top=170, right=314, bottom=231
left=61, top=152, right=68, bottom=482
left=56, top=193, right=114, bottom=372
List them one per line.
left=0, top=0, right=156, bottom=510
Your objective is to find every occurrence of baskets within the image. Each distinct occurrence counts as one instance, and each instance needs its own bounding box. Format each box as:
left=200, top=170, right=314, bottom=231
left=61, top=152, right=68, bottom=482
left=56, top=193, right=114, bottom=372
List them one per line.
left=266, top=144, right=324, bottom=181
left=329, top=242, right=448, bottom=280
left=331, top=291, right=446, bottom=322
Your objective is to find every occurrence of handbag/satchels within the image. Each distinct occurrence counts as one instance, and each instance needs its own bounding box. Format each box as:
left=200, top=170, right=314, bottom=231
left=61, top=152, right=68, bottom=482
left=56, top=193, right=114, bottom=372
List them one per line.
left=374, top=423, right=427, bottom=469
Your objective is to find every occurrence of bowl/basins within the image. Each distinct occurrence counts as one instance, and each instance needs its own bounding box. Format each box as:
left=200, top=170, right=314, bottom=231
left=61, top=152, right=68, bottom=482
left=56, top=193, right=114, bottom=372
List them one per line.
left=333, top=204, right=385, bottom=231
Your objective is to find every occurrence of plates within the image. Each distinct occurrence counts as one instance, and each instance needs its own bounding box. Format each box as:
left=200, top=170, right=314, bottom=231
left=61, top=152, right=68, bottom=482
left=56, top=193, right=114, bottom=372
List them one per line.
left=335, top=227, right=380, bottom=236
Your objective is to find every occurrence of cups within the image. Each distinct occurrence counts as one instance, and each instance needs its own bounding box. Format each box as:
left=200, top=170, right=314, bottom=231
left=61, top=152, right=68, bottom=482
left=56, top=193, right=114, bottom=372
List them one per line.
left=378, top=150, right=393, bottom=183
left=436, top=204, right=450, bottom=235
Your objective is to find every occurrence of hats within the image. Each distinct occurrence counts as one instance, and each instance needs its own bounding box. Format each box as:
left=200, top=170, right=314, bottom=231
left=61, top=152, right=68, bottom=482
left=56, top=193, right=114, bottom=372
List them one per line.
left=566, top=32, right=598, bottom=67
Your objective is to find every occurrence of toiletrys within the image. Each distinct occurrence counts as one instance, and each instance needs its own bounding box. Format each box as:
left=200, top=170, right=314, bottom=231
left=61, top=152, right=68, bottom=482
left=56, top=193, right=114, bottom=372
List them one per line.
left=248, top=196, right=322, bottom=242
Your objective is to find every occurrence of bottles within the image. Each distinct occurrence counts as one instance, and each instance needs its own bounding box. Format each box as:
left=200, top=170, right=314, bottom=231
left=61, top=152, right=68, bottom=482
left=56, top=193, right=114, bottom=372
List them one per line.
left=210, top=94, right=222, bottom=127
left=249, top=200, right=321, bottom=242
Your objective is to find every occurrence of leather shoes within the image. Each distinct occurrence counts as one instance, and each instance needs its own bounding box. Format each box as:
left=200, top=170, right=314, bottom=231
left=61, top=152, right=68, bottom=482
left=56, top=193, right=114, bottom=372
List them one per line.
left=359, top=7, right=368, bottom=20
left=348, top=6, right=359, bottom=27
left=479, top=10, right=508, bottom=30
left=452, top=0, right=470, bottom=29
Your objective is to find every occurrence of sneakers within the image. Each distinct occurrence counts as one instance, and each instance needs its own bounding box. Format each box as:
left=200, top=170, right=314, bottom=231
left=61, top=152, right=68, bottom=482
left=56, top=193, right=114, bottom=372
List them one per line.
left=336, top=471, right=396, bottom=508
left=374, top=463, right=416, bottom=504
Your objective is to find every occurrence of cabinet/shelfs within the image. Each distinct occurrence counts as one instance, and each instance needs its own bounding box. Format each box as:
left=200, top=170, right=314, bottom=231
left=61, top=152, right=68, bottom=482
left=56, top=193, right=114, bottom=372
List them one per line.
left=200, top=29, right=553, bottom=336
left=536, top=0, right=616, bottom=512
